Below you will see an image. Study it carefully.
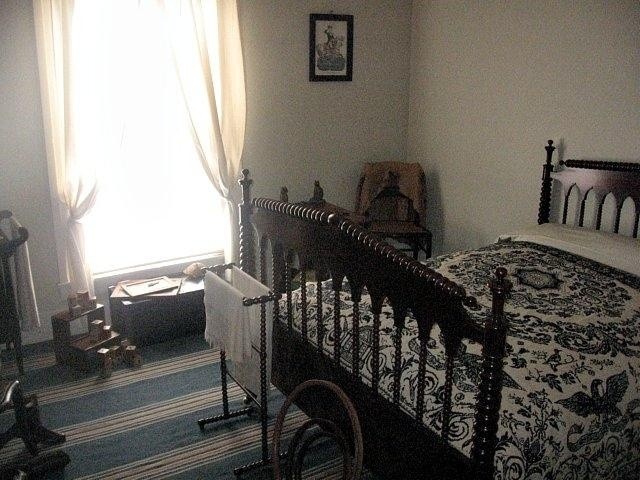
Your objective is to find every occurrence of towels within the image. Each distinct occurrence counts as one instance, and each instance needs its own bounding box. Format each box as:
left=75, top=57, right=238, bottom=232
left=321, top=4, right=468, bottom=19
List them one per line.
left=231, top=266, right=272, bottom=396
left=203, top=270, right=252, bottom=361
left=10, top=218, right=40, bottom=331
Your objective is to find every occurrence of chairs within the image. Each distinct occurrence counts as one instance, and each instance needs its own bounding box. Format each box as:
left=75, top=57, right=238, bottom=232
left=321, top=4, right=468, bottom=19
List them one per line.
left=364, top=161, right=432, bottom=261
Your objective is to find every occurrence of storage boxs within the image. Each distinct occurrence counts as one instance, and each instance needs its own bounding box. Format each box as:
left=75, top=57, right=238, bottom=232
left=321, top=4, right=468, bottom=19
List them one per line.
left=111, top=275, right=206, bottom=348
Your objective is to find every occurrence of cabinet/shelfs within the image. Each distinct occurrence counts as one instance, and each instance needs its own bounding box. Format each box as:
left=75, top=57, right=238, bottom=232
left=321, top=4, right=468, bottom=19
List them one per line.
left=198, top=264, right=290, bottom=474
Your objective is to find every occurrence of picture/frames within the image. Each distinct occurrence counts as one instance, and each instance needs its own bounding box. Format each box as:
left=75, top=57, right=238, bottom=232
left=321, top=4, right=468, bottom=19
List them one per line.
left=110, top=277, right=182, bottom=297
left=121, top=276, right=178, bottom=298
left=309, top=13, right=353, bottom=81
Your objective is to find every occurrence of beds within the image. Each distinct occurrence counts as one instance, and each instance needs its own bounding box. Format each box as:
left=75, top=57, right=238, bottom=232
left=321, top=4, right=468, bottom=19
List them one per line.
left=238, top=141, right=640, bottom=480
